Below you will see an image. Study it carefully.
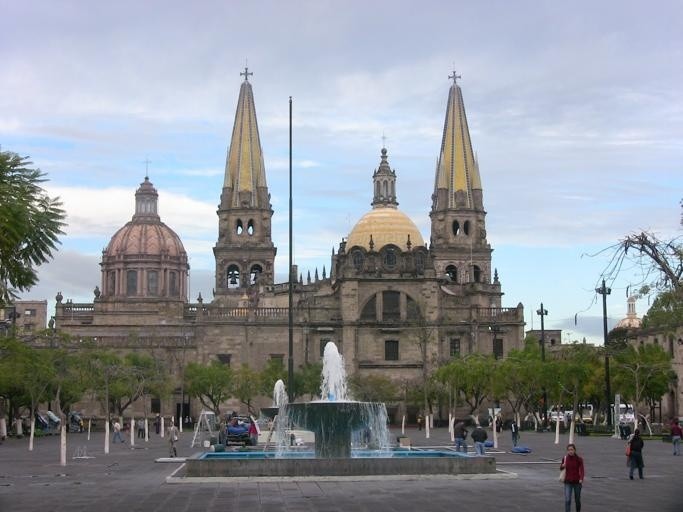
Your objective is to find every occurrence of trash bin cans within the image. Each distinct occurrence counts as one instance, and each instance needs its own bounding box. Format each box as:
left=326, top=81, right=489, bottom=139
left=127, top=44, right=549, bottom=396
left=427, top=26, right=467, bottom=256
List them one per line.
left=619, top=425, right=631, bottom=440
left=576, top=424, right=586, bottom=436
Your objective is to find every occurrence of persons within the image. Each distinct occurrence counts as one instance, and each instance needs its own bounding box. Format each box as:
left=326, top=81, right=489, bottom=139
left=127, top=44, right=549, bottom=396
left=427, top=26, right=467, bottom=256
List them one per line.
left=66, top=413, right=193, bottom=443
left=416, top=413, right=646, bottom=441
left=671, top=421, right=683, bottom=456
left=629, top=429, right=644, bottom=480
left=166, top=421, right=178, bottom=457
left=560, top=443, right=584, bottom=512
left=471, top=426, right=488, bottom=455
left=511, top=420, right=519, bottom=447
left=672, top=418, right=682, bottom=429
left=218, top=412, right=273, bottom=447
left=454, top=421, right=468, bottom=454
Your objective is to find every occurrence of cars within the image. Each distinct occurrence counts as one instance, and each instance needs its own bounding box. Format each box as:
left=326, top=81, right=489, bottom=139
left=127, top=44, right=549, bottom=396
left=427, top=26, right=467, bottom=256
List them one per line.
left=521, top=397, right=636, bottom=428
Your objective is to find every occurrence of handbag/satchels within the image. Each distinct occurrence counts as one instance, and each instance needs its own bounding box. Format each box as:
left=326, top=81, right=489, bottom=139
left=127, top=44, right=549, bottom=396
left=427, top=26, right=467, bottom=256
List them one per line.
left=559, top=469, right=567, bottom=482
left=625, top=445, right=630, bottom=456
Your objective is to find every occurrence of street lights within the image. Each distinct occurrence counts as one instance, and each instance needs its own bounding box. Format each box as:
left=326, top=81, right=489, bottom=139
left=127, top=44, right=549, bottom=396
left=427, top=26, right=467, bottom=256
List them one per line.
left=536, top=302, right=551, bottom=433
left=594, top=279, right=613, bottom=435
left=487, top=319, right=501, bottom=409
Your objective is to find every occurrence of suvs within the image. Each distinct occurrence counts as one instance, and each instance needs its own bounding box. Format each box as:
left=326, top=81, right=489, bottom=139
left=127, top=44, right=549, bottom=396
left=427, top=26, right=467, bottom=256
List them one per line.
left=217, top=413, right=260, bottom=446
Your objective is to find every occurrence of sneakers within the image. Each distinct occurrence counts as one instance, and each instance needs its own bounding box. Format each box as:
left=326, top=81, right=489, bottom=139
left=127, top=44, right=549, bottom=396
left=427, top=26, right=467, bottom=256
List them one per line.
left=629, top=475, right=645, bottom=480
